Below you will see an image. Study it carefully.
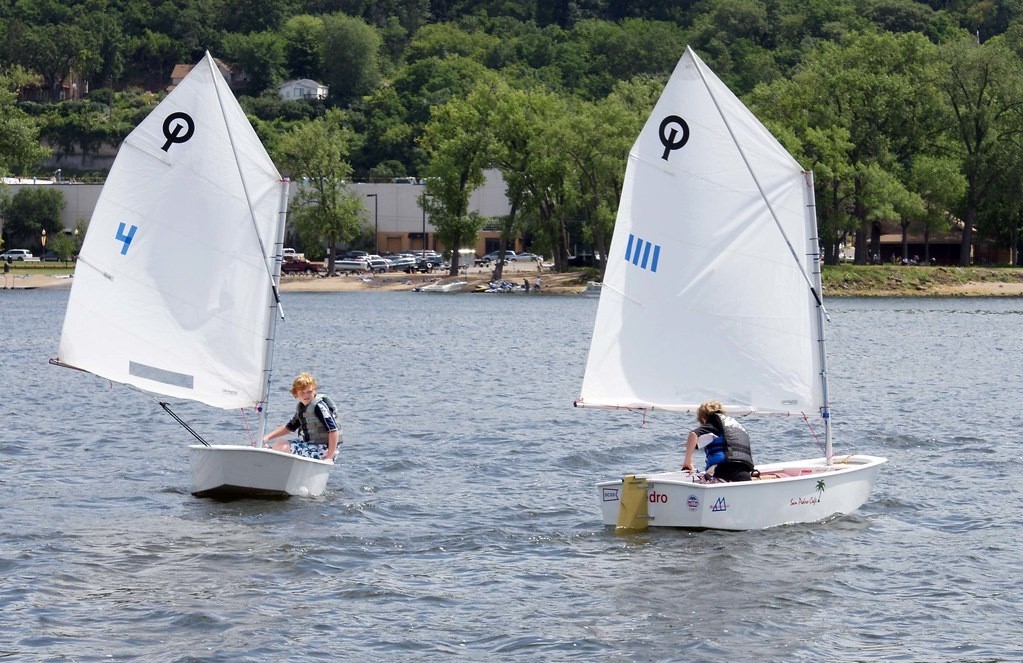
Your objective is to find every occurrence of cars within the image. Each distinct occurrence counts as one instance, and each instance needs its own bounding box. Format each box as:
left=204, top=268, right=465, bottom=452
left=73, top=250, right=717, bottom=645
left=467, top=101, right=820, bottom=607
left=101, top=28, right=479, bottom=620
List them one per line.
left=336, top=249, right=442, bottom=273
left=419, top=175, right=444, bottom=185
left=39, top=249, right=73, bottom=262
left=299, top=177, right=330, bottom=184
left=392, top=176, right=418, bottom=185
left=481, top=250, right=517, bottom=263
left=282, top=247, right=295, bottom=256
left=508, top=252, right=543, bottom=263
left=0, top=248, right=34, bottom=261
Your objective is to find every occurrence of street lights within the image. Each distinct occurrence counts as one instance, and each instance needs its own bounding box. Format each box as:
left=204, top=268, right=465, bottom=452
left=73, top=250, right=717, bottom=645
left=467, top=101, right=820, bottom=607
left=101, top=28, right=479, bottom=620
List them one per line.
left=422, top=192, right=436, bottom=258
left=367, top=193, right=378, bottom=254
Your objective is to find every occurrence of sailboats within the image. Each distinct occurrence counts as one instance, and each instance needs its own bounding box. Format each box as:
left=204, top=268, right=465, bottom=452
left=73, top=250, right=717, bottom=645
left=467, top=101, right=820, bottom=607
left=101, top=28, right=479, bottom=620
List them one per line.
left=50, top=48, right=336, bottom=505
left=574, top=43, right=889, bottom=529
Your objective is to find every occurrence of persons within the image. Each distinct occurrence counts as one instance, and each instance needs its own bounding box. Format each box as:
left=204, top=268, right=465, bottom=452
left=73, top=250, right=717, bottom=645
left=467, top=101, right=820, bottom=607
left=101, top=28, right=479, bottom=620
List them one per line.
left=523, top=277, right=530, bottom=293
left=681, top=401, right=754, bottom=482
left=537, top=254, right=544, bottom=268
left=534, top=276, right=543, bottom=294
left=263, top=371, right=342, bottom=462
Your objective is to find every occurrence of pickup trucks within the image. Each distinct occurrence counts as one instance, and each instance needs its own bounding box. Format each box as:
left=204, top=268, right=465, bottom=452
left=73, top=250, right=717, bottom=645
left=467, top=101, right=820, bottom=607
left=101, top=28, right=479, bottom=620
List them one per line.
left=281, top=256, right=324, bottom=275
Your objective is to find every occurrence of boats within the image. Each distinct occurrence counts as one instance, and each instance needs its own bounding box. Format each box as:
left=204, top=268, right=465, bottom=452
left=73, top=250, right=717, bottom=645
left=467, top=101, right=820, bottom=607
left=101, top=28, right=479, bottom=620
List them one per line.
left=412, top=277, right=468, bottom=292
left=325, top=257, right=368, bottom=271
